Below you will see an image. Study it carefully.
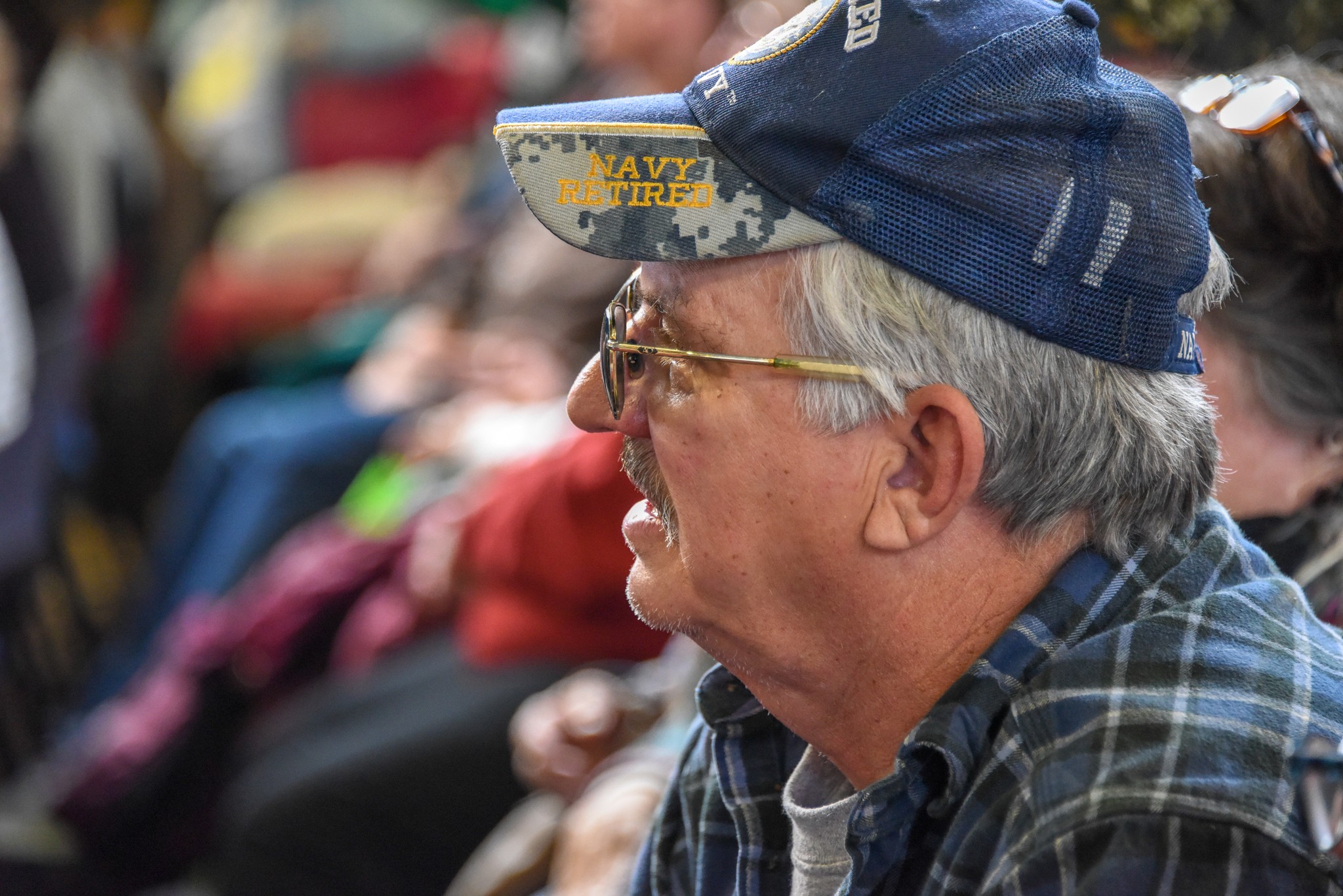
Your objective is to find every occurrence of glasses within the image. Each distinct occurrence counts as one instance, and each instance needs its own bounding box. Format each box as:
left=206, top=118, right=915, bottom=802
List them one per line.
left=1179, top=64, right=1343, bottom=197
left=599, top=263, right=929, bottom=422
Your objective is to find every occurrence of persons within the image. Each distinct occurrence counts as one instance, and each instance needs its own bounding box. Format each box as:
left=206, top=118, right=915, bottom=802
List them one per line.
left=1085, top=0, right=1343, bottom=89
left=493, top=0, right=1343, bottom=896
left=0, top=0, right=725, bottom=896
left=448, top=53, right=1343, bottom=896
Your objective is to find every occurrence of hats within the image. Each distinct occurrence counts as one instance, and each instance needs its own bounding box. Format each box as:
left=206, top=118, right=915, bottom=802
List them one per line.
left=490, top=0, right=1209, bottom=376
